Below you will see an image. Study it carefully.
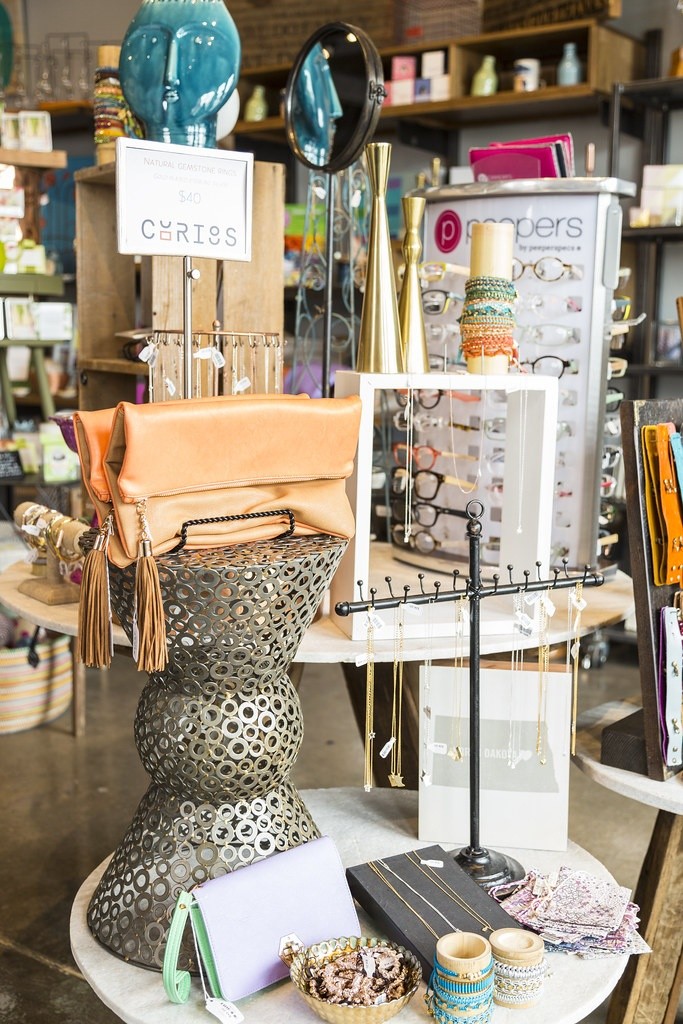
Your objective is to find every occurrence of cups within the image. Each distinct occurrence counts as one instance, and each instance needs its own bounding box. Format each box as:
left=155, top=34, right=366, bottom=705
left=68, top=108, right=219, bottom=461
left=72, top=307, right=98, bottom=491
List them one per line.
left=513, top=59, right=540, bottom=91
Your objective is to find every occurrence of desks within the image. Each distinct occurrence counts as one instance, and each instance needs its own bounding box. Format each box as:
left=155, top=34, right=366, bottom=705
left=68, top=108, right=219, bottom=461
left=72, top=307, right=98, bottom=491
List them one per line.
left=67, top=786, right=632, bottom=1024
left=0, top=551, right=634, bottom=792
left=571, top=692, right=683, bottom=1024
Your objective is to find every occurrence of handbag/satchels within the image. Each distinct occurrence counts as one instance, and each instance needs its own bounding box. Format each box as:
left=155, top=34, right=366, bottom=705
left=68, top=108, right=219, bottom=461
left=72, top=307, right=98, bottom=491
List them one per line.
left=72, top=391, right=363, bottom=672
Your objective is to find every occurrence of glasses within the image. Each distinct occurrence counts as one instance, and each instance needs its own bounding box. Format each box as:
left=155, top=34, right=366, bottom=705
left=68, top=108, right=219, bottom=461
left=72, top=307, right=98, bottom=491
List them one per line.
left=597, top=258, right=645, bottom=556
left=384, top=250, right=580, bottom=569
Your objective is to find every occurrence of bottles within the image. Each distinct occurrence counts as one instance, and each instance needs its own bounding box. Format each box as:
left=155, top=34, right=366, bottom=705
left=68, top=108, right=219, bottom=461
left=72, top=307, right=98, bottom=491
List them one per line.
left=559, top=44, right=583, bottom=87
left=246, top=86, right=267, bottom=121
left=470, top=55, right=497, bottom=96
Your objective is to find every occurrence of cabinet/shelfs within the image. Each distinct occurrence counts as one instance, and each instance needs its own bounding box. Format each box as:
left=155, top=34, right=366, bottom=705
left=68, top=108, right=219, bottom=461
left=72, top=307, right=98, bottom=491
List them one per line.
left=230, top=17, right=646, bottom=398
left=607, top=79, right=683, bottom=398
left=77, top=157, right=287, bottom=413
